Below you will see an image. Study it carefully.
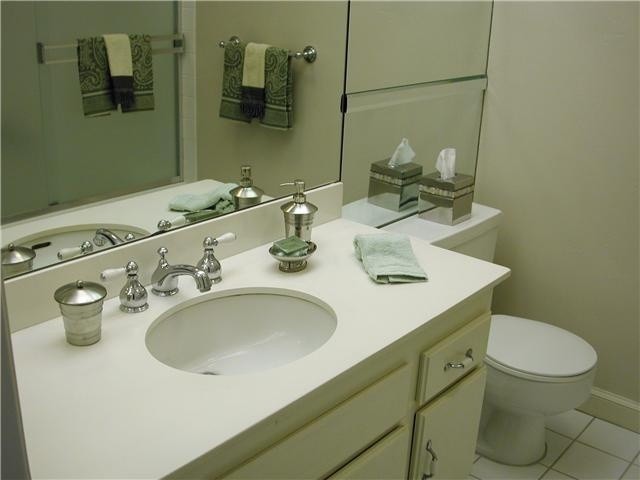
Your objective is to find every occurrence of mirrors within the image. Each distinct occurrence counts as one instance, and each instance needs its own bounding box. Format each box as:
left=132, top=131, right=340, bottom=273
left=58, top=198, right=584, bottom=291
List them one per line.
left=340, top=75, right=488, bottom=228
left=1, top=1, right=351, bottom=285
left=343, top=1, right=493, bottom=96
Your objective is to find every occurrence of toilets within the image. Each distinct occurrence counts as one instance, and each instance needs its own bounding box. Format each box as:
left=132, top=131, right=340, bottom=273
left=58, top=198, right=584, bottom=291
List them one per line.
left=374, top=203, right=597, bottom=465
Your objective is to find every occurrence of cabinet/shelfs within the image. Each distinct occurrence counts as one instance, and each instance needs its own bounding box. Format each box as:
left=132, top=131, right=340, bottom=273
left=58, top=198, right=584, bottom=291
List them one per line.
left=411, top=311, right=493, bottom=480
left=214, top=360, right=412, bottom=480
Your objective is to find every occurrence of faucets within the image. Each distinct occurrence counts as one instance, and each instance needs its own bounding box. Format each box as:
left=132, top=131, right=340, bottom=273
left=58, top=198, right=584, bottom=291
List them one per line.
left=93, top=229, right=135, bottom=247
left=150, top=246, right=212, bottom=297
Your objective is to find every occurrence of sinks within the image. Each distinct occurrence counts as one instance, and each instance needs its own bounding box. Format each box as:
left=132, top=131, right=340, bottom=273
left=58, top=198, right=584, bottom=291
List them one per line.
left=2, top=221, right=151, bottom=270
left=145, top=286, right=338, bottom=374
